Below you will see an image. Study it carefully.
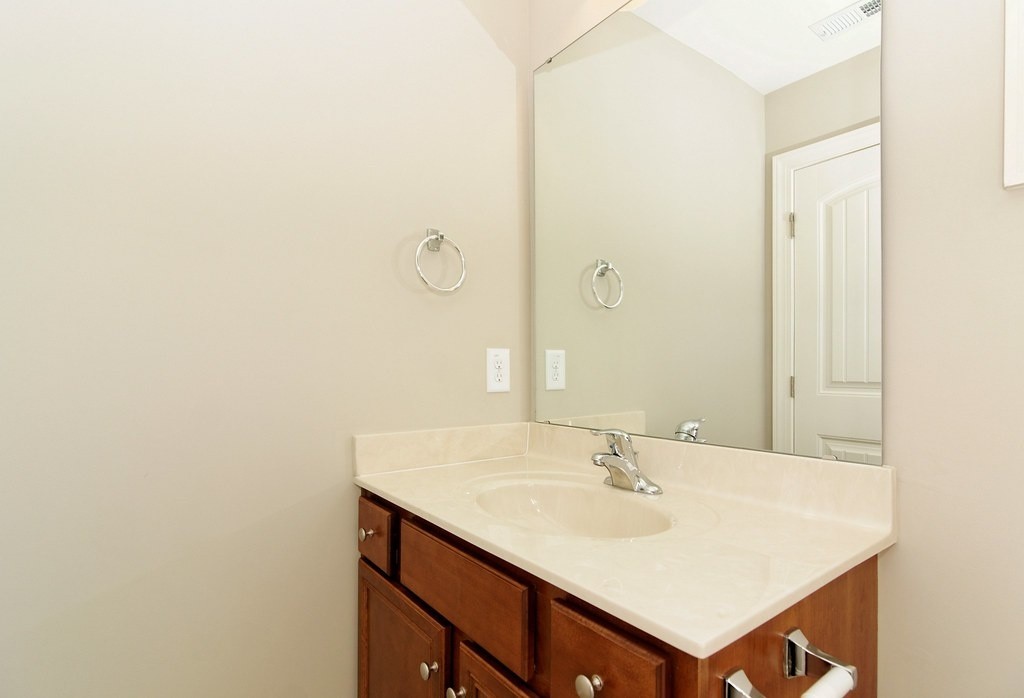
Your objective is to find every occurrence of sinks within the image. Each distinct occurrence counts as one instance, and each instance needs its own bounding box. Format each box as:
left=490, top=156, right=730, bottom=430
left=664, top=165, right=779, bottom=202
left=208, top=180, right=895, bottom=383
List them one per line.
left=464, top=467, right=685, bottom=547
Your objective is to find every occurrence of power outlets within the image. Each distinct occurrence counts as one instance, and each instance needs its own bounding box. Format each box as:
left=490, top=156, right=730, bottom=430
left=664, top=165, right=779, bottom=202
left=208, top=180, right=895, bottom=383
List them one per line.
left=486, top=348, right=510, bottom=392
left=545, top=350, right=565, bottom=389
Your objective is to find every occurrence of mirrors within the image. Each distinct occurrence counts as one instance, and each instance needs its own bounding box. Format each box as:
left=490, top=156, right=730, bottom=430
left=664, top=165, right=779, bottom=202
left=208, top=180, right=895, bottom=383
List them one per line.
left=532, top=0, right=882, bottom=467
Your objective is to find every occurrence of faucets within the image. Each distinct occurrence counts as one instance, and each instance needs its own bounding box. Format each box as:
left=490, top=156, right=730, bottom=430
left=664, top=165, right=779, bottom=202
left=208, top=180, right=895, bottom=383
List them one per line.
left=673, top=415, right=709, bottom=442
left=587, top=428, right=641, bottom=492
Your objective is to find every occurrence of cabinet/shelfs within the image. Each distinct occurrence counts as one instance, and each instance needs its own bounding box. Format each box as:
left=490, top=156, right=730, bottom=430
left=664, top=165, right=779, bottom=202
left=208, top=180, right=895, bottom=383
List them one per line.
left=358, top=496, right=878, bottom=698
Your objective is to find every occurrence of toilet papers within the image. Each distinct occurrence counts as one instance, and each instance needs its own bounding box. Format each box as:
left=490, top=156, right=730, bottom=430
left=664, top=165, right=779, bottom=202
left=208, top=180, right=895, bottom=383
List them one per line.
left=800, top=665, right=855, bottom=698
